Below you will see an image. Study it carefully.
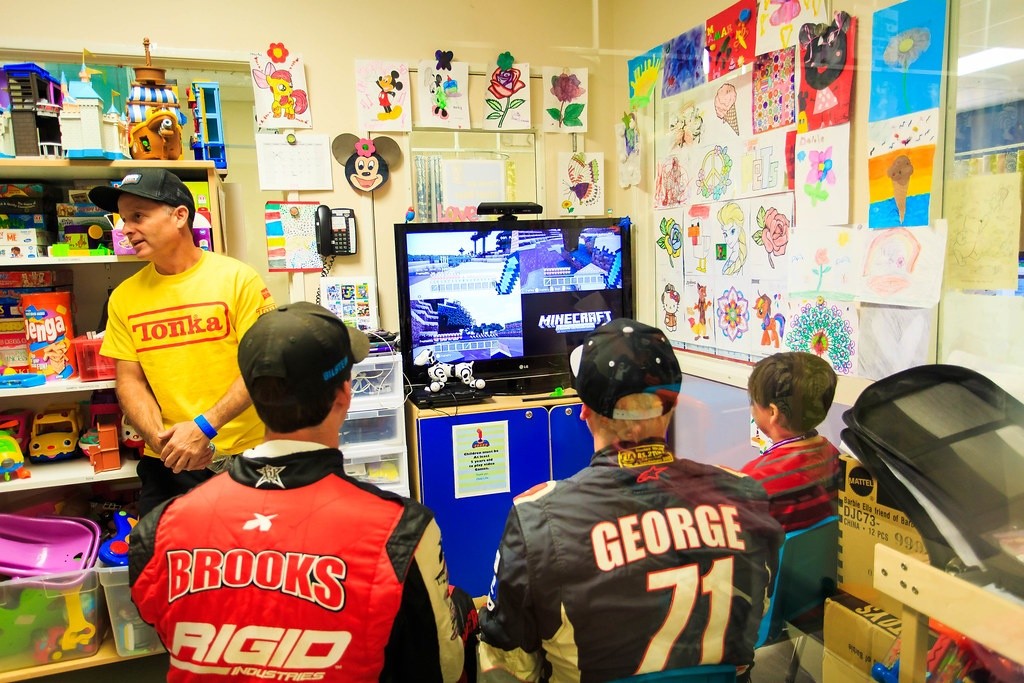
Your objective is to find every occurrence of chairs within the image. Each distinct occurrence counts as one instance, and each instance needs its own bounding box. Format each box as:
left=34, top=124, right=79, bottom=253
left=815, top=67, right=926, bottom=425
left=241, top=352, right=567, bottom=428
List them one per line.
left=753, top=514, right=838, bottom=683
left=608, top=664, right=736, bottom=683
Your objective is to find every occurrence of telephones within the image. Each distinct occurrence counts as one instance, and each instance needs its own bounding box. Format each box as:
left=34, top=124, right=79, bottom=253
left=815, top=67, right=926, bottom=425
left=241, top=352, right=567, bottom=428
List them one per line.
left=314, top=204, right=359, bottom=257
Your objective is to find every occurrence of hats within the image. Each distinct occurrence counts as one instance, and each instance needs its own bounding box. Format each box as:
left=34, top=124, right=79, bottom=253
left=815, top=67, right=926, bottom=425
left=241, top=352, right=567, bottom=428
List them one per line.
left=88, top=168, right=195, bottom=217
left=569, top=318, right=683, bottom=420
left=238, top=300, right=370, bottom=407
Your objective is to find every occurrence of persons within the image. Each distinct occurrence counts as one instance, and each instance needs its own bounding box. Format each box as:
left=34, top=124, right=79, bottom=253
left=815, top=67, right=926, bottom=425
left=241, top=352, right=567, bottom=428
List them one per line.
left=718, top=352, right=842, bottom=615
left=478, top=317, right=786, bottom=683
left=89, top=167, right=278, bottom=515
left=129, top=301, right=480, bottom=683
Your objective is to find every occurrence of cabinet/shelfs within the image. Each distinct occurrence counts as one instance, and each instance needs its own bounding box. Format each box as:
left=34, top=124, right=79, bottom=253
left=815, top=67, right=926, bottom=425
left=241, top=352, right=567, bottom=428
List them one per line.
left=873, top=543, right=1024, bottom=683
left=404, top=387, right=593, bottom=610
left=0, top=154, right=228, bottom=683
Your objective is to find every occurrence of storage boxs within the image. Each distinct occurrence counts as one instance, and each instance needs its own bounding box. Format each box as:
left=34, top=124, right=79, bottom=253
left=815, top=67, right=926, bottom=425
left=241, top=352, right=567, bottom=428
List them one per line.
left=0, top=180, right=213, bottom=382
left=834, top=451, right=955, bottom=619
left=93, top=553, right=158, bottom=658
left=0, top=560, right=107, bottom=673
left=339, top=355, right=411, bottom=499
left=821, top=592, right=939, bottom=683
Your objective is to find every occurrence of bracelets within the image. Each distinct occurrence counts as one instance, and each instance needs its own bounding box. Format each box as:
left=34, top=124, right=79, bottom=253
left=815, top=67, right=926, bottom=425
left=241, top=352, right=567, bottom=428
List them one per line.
left=194, top=415, right=218, bottom=440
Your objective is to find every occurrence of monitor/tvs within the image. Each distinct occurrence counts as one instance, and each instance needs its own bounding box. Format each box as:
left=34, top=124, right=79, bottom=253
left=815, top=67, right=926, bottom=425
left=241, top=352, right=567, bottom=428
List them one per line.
left=394, top=218, right=632, bottom=397
